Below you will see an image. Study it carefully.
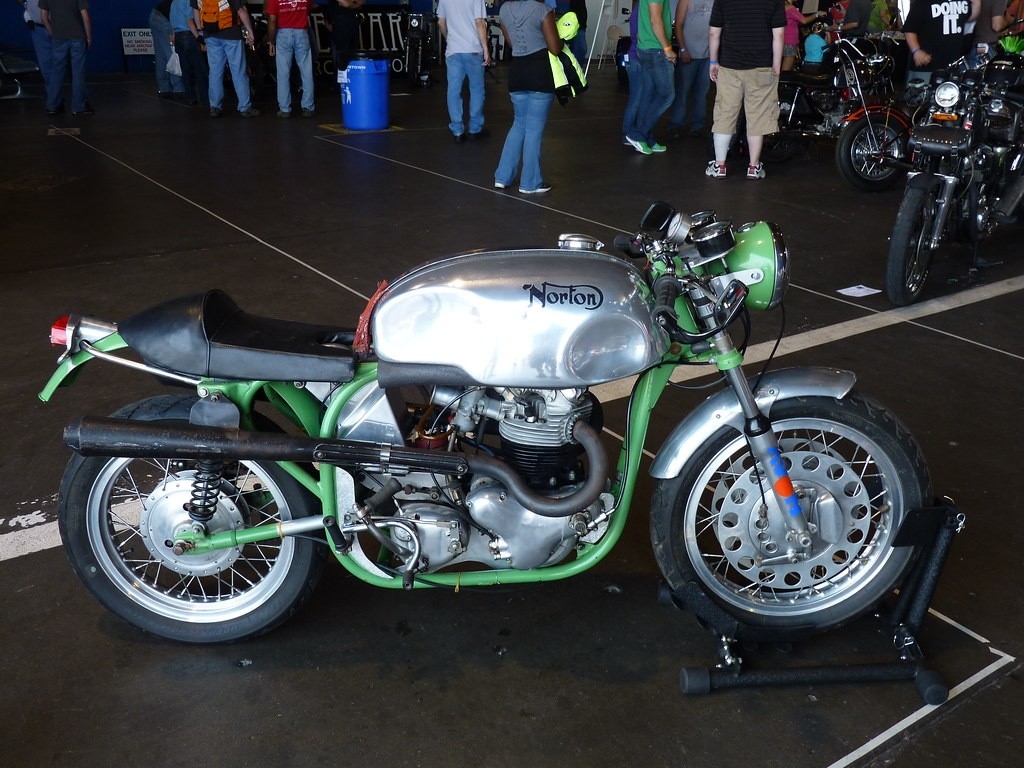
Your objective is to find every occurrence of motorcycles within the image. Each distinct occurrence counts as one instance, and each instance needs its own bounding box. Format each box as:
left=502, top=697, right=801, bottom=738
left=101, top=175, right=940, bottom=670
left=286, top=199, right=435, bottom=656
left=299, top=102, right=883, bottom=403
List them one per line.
left=615, top=7, right=681, bottom=89
left=738, top=26, right=916, bottom=193
left=37, top=200, right=937, bottom=651
left=884, top=54, right=1024, bottom=309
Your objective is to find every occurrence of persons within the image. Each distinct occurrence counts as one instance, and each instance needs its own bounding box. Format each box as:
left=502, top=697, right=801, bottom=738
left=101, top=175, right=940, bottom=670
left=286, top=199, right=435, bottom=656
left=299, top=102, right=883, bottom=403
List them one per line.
left=263, top=0, right=316, bottom=118
left=555, top=0, right=588, bottom=67
left=24, top=0, right=95, bottom=115
left=991, top=0, right=1024, bottom=36
left=494, top=0, right=565, bottom=193
left=622, top=0, right=714, bottom=155
left=436, top=0, right=491, bottom=142
left=327, top=0, right=366, bottom=71
left=705, top=0, right=787, bottom=180
left=149, top=0, right=261, bottom=118
left=779, top=0, right=981, bottom=95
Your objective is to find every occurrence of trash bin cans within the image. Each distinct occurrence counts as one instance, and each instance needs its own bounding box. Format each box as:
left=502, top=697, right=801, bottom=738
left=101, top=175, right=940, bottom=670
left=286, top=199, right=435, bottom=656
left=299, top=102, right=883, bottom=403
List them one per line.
left=335, top=50, right=391, bottom=131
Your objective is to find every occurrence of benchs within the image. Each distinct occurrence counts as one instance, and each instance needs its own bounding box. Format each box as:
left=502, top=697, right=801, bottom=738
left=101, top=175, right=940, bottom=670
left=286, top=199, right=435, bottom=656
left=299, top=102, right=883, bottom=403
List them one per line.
left=0, top=53, right=37, bottom=99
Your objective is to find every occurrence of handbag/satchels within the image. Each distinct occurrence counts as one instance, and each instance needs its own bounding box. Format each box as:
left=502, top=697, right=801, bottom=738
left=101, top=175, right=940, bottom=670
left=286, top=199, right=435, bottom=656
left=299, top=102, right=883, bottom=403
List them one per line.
left=166, top=45, right=183, bottom=76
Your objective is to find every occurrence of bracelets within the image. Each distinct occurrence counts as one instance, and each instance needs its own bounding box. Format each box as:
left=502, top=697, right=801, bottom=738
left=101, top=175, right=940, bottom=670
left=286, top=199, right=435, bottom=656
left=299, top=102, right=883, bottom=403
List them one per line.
left=664, top=45, right=672, bottom=52
left=710, top=60, right=718, bottom=63
left=680, top=48, right=687, bottom=51
left=815, top=12, right=820, bottom=17
left=912, top=48, right=923, bottom=56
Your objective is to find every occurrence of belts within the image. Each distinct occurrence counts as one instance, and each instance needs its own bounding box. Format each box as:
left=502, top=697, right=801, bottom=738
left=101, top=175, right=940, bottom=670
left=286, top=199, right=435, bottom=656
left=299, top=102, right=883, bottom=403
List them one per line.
left=35, top=23, right=45, bottom=28
left=175, top=31, right=192, bottom=36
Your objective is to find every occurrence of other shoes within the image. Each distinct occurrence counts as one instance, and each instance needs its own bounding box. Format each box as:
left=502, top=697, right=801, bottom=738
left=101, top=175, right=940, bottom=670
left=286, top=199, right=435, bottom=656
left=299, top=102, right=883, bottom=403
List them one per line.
left=48, top=110, right=63, bottom=116
left=469, top=128, right=490, bottom=140
left=454, top=134, right=467, bottom=144
left=237, top=107, right=252, bottom=115
left=201, top=101, right=209, bottom=105
left=665, top=129, right=680, bottom=140
left=301, top=108, right=317, bottom=118
left=210, top=108, right=220, bottom=117
left=691, top=128, right=709, bottom=137
left=164, top=93, right=173, bottom=100
left=185, top=103, right=200, bottom=109
left=172, top=93, right=185, bottom=101
left=278, top=111, right=290, bottom=119
left=623, top=138, right=631, bottom=145
left=72, top=108, right=96, bottom=118
left=328, top=88, right=342, bottom=97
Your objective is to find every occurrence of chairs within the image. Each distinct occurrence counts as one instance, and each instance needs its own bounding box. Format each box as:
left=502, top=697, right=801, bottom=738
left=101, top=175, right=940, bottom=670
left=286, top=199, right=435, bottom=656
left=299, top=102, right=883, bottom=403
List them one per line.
left=601, top=24, right=626, bottom=71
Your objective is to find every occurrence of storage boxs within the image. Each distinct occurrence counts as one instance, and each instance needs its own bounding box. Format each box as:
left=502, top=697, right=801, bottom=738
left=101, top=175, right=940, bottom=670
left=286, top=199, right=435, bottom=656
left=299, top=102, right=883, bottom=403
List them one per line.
left=984, top=53, right=1024, bottom=88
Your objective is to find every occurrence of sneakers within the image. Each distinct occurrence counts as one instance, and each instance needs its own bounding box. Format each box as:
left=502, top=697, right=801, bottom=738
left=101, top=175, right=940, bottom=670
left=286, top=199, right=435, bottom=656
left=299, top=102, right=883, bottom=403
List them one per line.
left=704, top=160, right=726, bottom=179
left=626, top=135, right=653, bottom=155
left=494, top=181, right=505, bottom=188
left=747, top=162, right=766, bottom=180
left=651, top=143, right=666, bottom=153
left=518, top=183, right=552, bottom=194
